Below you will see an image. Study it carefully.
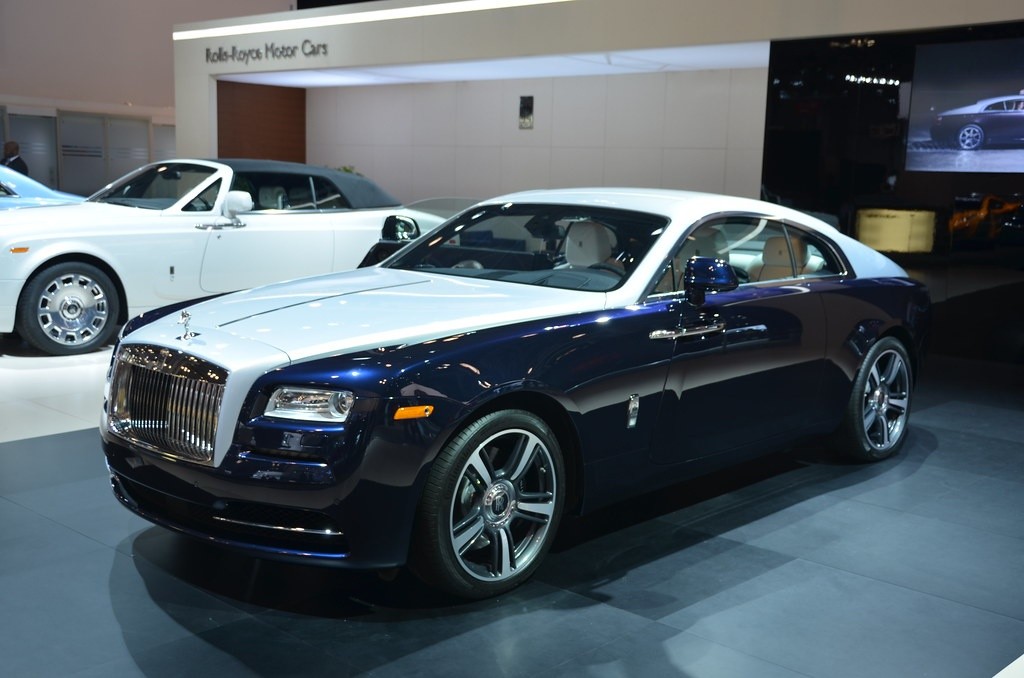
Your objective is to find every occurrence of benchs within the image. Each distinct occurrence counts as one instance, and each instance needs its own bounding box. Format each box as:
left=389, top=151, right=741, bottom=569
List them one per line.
left=729, top=234, right=826, bottom=283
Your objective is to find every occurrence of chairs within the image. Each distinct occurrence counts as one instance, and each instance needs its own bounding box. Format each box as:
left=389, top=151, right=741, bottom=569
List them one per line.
left=659, top=226, right=746, bottom=292
left=259, top=184, right=291, bottom=210
left=551, top=222, right=624, bottom=276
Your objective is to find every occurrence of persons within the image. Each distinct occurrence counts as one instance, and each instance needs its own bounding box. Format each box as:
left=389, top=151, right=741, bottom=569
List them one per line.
left=0, top=141, right=28, bottom=176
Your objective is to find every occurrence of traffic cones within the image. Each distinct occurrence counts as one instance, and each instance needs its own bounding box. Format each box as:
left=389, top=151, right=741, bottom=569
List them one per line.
left=941, top=194, right=1024, bottom=255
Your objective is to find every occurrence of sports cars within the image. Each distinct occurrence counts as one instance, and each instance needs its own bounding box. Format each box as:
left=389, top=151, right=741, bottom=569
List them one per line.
left=0, top=157, right=458, bottom=361
left=99, top=187, right=932, bottom=602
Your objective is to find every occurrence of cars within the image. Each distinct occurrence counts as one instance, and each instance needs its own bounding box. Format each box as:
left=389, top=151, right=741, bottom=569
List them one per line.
left=929, top=94, right=1024, bottom=152
left=0, top=164, right=91, bottom=209
